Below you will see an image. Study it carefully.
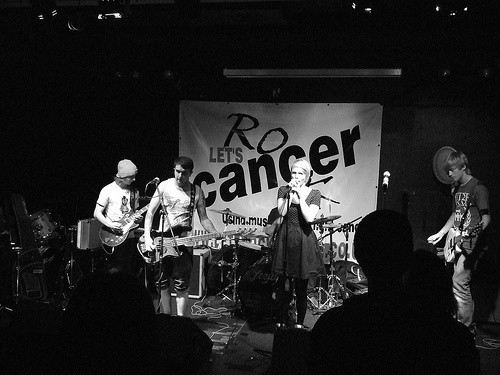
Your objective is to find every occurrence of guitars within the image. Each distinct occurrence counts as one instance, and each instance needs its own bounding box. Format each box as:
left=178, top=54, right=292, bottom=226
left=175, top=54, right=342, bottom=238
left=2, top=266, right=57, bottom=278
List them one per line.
left=137, top=225, right=239, bottom=266
left=443, top=221, right=484, bottom=263
left=98, top=203, right=150, bottom=248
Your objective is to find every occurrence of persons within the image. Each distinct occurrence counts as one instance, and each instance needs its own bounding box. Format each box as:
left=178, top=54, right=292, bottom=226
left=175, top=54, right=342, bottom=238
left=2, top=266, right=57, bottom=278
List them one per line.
left=427, top=152, right=491, bottom=327
left=264, top=207, right=283, bottom=235
left=144, top=156, right=223, bottom=316
left=270, top=158, right=321, bottom=327
left=93, top=159, right=143, bottom=270
left=0, top=210, right=481, bottom=375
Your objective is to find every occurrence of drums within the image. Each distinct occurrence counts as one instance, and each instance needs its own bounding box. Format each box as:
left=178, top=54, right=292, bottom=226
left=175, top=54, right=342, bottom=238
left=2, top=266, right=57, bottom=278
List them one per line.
left=10, top=208, right=78, bottom=304
left=225, top=241, right=288, bottom=318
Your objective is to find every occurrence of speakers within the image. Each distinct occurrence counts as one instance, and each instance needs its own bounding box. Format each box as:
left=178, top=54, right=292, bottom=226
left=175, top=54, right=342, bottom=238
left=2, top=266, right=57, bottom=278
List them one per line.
left=146, top=313, right=214, bottom=371
left=269, top=328, right=313, bottom=374
left=172, top=248, right=211, bottom=299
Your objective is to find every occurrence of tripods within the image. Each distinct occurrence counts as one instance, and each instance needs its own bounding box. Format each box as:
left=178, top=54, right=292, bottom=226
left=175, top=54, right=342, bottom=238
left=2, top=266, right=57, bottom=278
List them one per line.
left=203, top=237, right=253, bottom=308
left=305, top=196, right=351, bottom=313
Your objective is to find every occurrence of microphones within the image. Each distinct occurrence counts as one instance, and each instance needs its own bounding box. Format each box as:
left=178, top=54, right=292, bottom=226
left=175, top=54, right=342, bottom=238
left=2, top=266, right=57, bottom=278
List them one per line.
left=382, top=171, right=390, bottom=188
left=149, top=177, right=160, bottom=184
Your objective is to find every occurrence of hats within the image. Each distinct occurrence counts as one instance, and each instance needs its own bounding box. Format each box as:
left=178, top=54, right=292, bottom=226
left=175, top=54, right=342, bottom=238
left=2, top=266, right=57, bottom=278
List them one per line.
left=116, top=159, right=138, bottom=178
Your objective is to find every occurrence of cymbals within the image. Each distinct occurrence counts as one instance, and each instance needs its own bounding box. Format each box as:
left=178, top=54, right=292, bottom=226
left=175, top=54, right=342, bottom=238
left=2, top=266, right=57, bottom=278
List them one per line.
left=207, top=208, right=246, bottom=219
left=308, top=215, right=342, bottom=224
left=320, top=223, right=343, bottom=228
